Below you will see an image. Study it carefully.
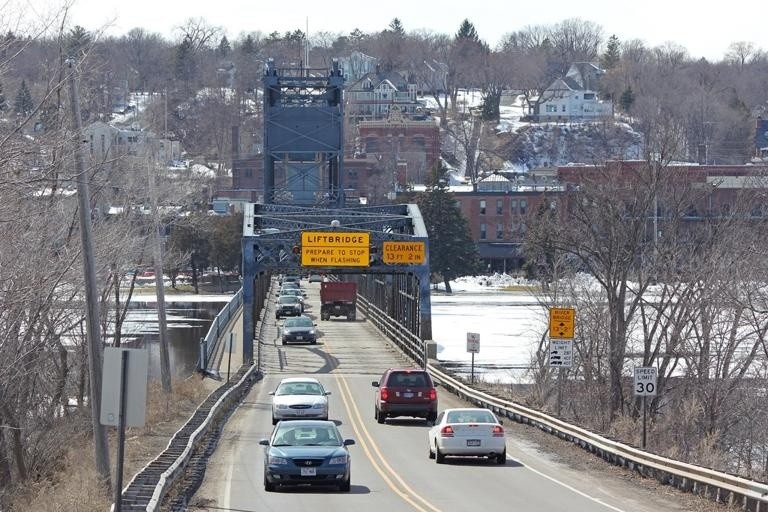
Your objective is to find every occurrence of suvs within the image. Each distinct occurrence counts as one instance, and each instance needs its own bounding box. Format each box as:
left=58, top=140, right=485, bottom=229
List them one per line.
left=370, top=368, right=439, bottom=425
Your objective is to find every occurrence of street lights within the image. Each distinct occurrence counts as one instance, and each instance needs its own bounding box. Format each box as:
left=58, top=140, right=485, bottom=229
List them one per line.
left=650, top=150, right=663, bottom=274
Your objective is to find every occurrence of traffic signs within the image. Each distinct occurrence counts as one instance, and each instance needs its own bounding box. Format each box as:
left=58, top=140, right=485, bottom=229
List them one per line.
left=382, top=242, right=426, bottom=266
left=633, top=365, right=657, bottom=398
left=548, top=339, right=573, bottom=367
left=548, top=305, right=574, bottom=338
left=464, top=332, right=480, bottom=354
left=299, top=231, right=371, bottom=267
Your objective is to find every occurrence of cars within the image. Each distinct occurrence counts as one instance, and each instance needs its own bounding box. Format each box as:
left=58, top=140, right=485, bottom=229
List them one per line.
left=91, top=264, right=240, bottom=290
left=262, top=269, right=357, bottom=494
left=426, top=407, right=509, bottom=468
